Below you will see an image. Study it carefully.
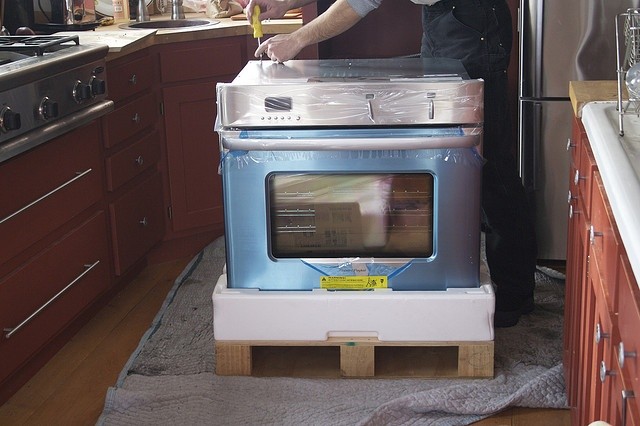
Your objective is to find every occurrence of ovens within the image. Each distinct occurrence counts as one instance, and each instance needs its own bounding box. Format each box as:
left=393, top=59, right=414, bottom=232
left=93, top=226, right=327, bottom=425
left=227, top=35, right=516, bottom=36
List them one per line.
left=214, top=58, right=485, bottom=287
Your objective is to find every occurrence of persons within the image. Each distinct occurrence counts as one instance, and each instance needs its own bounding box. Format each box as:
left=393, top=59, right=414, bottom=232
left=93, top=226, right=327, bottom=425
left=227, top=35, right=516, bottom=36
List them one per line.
left=239, top=0, right=537, bottom=329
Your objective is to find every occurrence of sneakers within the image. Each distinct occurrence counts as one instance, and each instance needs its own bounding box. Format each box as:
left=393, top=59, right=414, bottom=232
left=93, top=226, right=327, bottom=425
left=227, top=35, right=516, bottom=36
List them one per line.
left=495, top=292, right=533, bottom=327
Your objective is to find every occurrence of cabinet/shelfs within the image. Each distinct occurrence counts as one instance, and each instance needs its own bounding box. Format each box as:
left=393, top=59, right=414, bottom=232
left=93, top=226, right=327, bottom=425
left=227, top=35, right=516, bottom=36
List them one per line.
left=162, top=39, right=249, bottom=236
left=1, top=102, right=118, bottom=392
left=104, top=44, right=163, bottom=293
left=562, top=107, right=639, bottom=426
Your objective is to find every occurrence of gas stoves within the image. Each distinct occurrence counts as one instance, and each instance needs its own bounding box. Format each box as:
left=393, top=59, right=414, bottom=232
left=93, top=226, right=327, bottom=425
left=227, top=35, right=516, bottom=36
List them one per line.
left=0, top=32, right=110, bottom=144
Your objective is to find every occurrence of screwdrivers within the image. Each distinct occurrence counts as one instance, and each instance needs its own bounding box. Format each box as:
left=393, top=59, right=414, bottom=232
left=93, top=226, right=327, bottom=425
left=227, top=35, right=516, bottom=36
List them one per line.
left=252, top=6, right=262, bottom=66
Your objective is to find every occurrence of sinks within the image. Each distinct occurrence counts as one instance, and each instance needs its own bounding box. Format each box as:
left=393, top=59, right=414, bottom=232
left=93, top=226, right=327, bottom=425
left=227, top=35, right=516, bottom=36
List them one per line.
left=118, top=19, right=221, bottom=32
